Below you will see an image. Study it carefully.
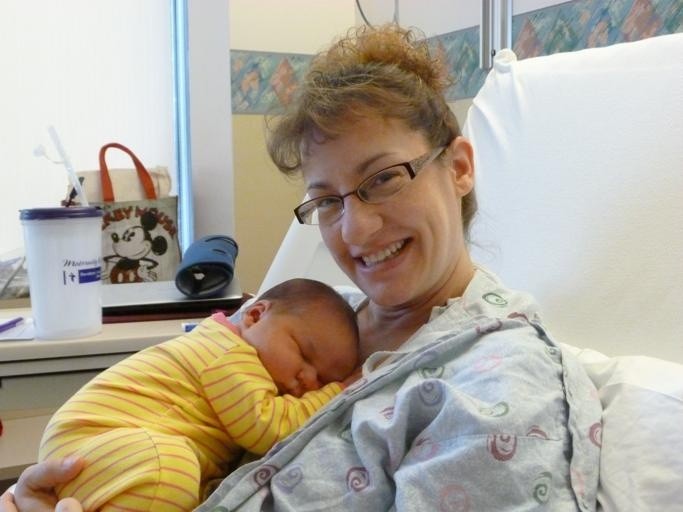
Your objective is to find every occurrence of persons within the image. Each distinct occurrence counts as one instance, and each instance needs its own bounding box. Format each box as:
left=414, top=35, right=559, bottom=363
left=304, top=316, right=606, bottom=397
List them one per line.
left=1, top=22, right=603, bottom=512
left=39, top=278, right=359, bottom=512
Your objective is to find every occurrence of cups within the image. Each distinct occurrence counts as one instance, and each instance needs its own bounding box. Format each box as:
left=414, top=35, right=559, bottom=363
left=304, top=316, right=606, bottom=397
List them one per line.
left=19, top=206, right=104, bottom=343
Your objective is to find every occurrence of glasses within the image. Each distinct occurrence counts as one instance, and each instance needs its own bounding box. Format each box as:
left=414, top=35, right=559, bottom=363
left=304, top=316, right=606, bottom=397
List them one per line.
left=290, top=146, right=448, bottom=226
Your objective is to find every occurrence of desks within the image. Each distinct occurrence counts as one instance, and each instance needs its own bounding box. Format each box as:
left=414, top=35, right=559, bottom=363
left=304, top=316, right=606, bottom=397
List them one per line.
left=1, top=297, right=203, bottom=481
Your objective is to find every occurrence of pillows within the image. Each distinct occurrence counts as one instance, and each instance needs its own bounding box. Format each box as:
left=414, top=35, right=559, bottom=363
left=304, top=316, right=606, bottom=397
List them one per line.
left=460, top=33, right=682, bottom=362
left=255, top=195, right=368, bottom=313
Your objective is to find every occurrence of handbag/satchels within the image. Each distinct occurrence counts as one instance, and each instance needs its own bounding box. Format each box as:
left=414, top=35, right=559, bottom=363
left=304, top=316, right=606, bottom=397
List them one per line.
left=88, top=195, right=181, bottom=285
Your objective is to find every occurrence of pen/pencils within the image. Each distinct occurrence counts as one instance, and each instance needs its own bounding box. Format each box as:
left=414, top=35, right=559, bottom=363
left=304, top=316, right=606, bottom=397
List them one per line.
left=0, top=317, right=24, bottom=333
